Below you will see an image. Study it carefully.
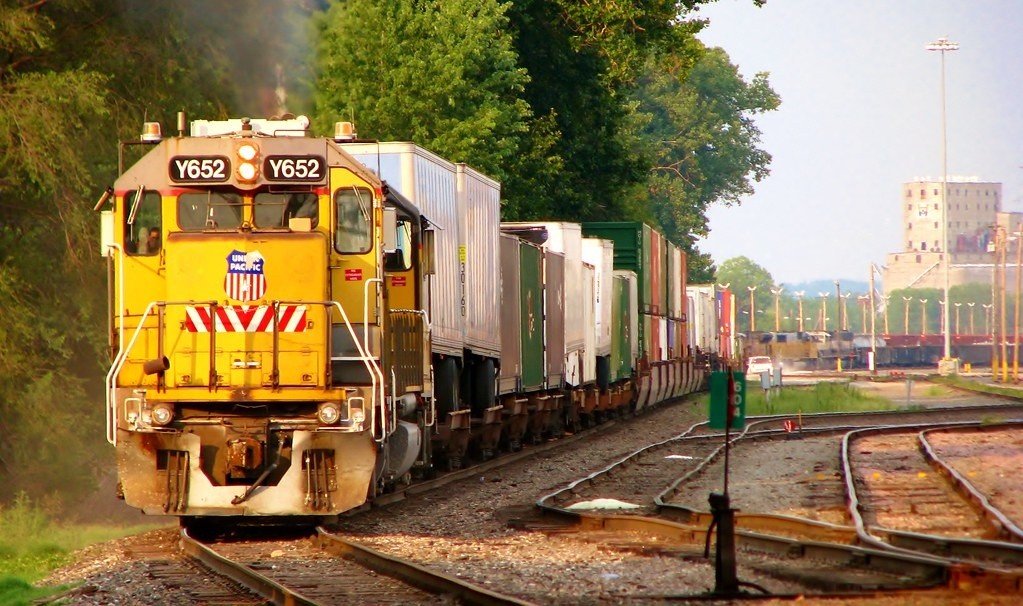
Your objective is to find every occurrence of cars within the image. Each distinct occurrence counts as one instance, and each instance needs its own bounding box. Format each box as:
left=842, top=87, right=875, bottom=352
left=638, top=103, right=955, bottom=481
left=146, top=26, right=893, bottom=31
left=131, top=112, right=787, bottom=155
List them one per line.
left=747, top=356, right=774, bottom=378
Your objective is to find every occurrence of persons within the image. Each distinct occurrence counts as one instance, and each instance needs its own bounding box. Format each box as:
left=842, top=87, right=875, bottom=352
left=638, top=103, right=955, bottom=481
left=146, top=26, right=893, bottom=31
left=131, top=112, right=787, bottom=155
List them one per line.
left=136, top=227, right=158, bottom=252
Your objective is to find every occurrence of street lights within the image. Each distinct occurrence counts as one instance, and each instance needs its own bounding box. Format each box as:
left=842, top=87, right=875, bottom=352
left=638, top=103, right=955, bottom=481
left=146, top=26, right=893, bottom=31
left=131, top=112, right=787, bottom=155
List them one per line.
left=793, top=290, right=804, bottom=332
left=919, top=298, right=928, bottom=335
left=858, top=295, right=868, bottom=335
left=982, top=304, right=992, bottom=337
left=747, top=285, right=758, bottom=333
left=902, top=296, right=913, bottom=335
left=839, top=293, right=851, bottom=330
left=954, top=303, right=962, bottom=335
left=879, top=295, right=891, bottom=335
left=818, top=292, right=830, bottom=332
left=938, top=300, right=945, bottom=336
left=925, top=36, right=960, bottom=378
left=968, top=302, right=976, bottom=335
left=770, top=288, right=783, bottom=333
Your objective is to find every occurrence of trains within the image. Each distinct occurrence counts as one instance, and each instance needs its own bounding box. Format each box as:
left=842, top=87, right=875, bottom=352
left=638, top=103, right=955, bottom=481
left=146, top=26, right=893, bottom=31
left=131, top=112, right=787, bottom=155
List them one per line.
left=92, top=108, right=1023, bottom=539
